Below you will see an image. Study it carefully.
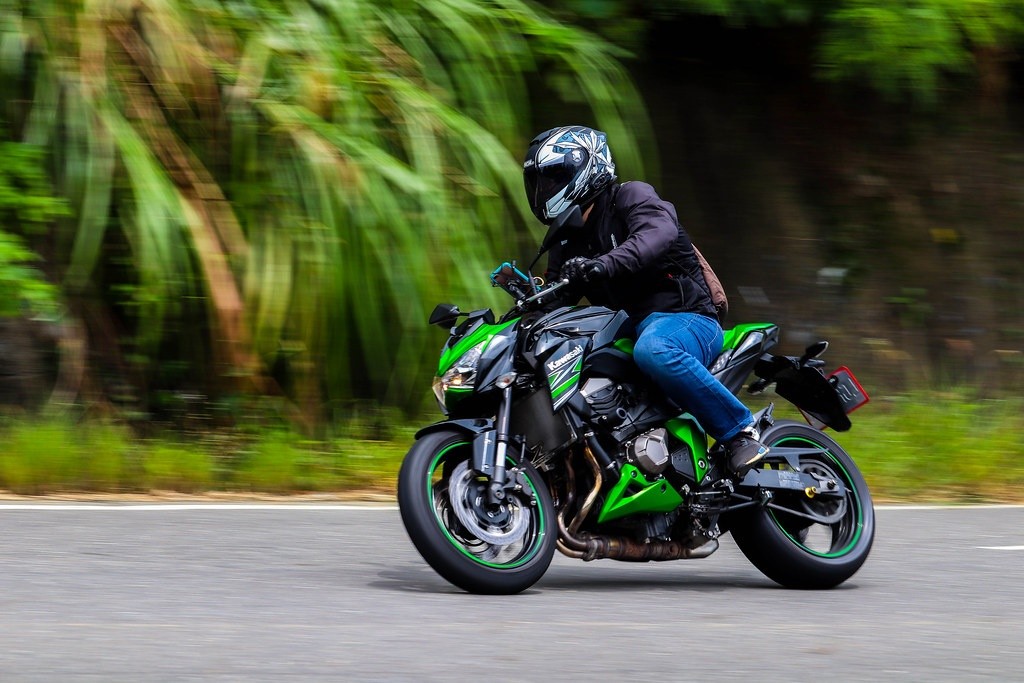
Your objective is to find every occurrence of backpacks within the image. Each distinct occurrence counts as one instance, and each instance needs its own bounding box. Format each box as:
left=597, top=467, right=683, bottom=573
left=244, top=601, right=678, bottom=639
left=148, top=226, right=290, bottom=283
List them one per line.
left=661, top=241, right=729, bottom=322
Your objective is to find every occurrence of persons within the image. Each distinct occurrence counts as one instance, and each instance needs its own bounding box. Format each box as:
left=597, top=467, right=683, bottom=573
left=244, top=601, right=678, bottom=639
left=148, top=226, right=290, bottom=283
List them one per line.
left=521, top=124, right=770, bottom=476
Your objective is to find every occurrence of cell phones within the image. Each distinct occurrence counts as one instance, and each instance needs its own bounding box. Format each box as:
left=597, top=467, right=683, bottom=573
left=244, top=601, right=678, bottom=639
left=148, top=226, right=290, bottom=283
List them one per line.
left=489, top=262, right=541, bottom=298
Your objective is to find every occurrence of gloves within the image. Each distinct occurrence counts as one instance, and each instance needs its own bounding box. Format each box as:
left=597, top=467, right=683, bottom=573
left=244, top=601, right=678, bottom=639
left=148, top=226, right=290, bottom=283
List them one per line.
left=560, top=256, right=607, bottom=282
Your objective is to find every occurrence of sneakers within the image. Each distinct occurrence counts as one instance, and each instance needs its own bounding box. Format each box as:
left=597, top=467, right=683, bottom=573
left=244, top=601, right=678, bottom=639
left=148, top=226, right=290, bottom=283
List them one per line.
left=725, top=425, right=770, bottom=472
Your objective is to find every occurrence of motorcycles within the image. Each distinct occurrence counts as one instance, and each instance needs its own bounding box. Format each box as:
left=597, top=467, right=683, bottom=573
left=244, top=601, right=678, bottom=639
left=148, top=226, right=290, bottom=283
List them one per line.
left=398, top=259, right=877, bottom=596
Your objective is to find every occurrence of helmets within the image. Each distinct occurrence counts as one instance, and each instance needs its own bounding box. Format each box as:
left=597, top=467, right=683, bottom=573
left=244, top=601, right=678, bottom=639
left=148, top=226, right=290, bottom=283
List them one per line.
left=523, top=126, right=618, bottom=225
